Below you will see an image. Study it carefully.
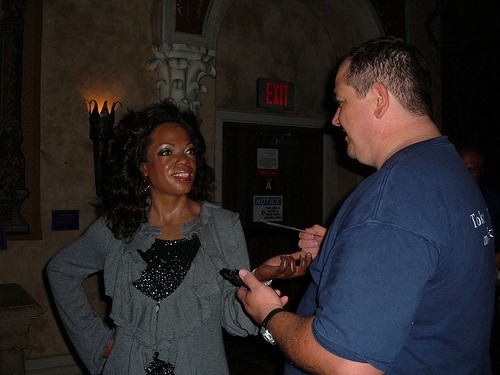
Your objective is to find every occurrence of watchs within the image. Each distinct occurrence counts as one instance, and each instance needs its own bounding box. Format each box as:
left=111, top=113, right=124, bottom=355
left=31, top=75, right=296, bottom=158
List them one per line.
left=261, top=307, right=283, bottom=347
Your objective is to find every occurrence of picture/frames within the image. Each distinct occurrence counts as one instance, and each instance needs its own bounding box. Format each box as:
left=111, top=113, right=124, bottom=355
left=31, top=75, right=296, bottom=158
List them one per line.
left=0, top=0, right=44, bottom=242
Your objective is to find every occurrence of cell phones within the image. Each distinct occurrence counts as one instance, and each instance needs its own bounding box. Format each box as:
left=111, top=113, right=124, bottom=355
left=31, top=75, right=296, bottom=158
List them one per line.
left=219, top=268, right=248, bottom=288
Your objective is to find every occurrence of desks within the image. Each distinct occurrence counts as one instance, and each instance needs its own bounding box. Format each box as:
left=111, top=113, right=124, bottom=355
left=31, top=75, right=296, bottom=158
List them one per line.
left=0, top=282, right=45, bottom=375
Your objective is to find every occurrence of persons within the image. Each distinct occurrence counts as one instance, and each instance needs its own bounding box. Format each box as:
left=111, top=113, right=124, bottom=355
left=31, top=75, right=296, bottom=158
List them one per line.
left=46, top=99, right=314, bottom=375
left=237, top=37, right=496, bottom=375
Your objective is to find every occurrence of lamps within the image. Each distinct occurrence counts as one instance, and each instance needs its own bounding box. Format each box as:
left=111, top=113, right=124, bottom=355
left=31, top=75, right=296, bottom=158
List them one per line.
left=84, top=97, right=124, bottom=197
